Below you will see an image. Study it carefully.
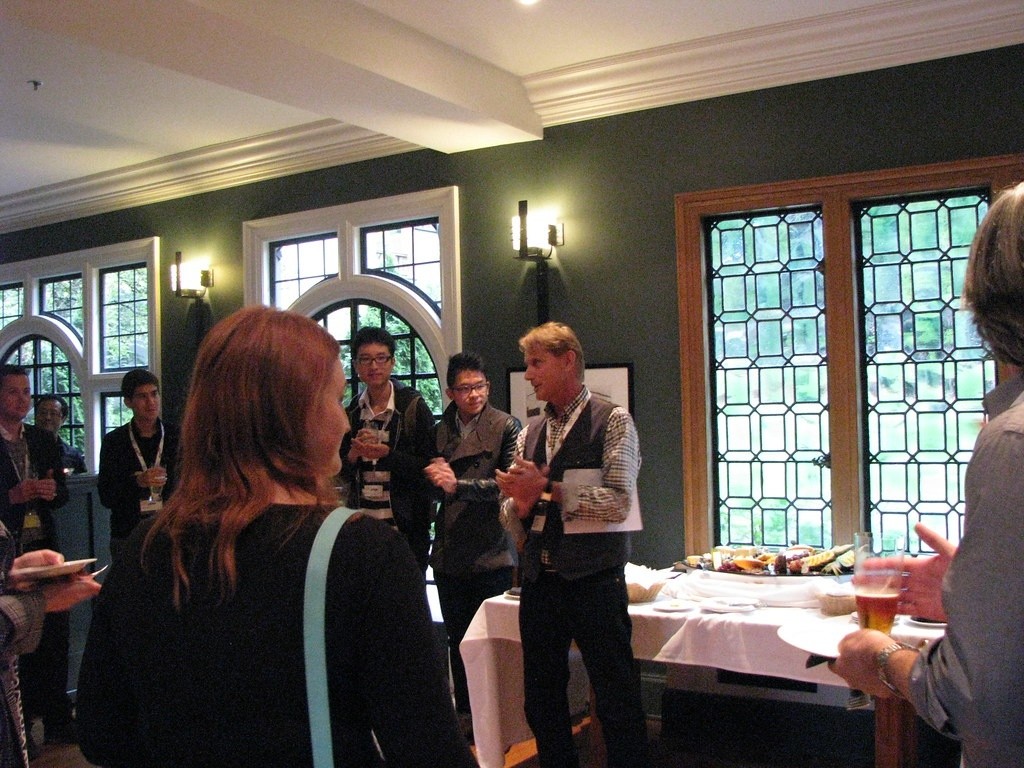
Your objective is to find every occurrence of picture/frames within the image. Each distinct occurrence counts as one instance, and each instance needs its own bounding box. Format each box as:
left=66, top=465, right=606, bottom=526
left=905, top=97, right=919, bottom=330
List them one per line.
left=505, top=361, right=635, bottom=430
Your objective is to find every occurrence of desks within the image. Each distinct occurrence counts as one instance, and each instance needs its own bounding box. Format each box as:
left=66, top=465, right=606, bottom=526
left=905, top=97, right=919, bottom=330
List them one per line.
left=458, top=570, right=948, bottom=768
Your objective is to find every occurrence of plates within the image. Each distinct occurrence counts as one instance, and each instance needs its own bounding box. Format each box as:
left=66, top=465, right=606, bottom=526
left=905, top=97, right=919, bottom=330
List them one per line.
left=776, top=621, right=860, bottom=659
left=906, top=612, right=947, bottom=630
left=9, top=559, right=97, bottom=581
left=653, top=601, right=693, bottom=612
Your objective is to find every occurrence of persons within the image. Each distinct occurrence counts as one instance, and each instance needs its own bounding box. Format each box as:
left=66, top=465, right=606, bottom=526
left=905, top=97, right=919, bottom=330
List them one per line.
left=0, top=518, right=102, bottom=768
left=97, top=368, right=184, bottom=560
left=494, top=321, right=650, bottom=768
left=0, top=364, right=78, bottom=761
left=422, top=351, right=523, bottom=744
left=826, top=176, right=1024, bottom=768
left=338, top=326, right=435, bottom=579
left=34, top=394, right=88, bottom=475
left=71, top=306, right=478, bottom=768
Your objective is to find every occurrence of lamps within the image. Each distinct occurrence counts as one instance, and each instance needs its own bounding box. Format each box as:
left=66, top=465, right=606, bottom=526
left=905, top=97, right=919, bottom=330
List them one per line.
left=170, top=250, right=215, bottom=345
left=509, top=200, right=565, bottom=326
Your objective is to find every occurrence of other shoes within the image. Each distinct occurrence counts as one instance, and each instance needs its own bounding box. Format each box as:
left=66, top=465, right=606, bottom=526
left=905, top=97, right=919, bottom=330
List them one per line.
left=43, top=715, right=79, bottom=744
left=24, top=730, right=40, bottom=762
left=460, top=717, right=474, bottom=745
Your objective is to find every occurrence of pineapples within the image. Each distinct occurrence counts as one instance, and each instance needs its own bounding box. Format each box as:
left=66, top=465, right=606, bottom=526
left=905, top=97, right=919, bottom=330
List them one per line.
left=800, top=543, right=856, bottom=575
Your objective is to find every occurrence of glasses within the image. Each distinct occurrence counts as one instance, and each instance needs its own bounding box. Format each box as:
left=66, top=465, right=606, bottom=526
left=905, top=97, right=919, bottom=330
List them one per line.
left=450, top=381, right=490, bottom=394
left=38, top=410, right=62, bottom=418
left=351, top=354, right=395, bottom=366
left=130, top=390, right=160, bottom=400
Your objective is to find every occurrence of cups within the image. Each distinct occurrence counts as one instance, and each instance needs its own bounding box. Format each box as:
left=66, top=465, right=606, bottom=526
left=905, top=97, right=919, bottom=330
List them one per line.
left=853, top=531, right=906, bottom=633
left=363, top=422, right=379, bottom=446
left=23, top=470, right=38, bottom=501
left=150, top=463, right=167, bottom=503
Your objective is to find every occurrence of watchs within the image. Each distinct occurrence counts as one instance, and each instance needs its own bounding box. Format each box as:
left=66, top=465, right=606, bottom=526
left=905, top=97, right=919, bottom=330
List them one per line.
left=877, top=643, right=920, bottom=700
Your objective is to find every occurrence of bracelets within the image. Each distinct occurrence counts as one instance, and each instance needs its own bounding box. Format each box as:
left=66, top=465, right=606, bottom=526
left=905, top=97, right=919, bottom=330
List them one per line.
left=543, top=479, right=554, bottom=494
